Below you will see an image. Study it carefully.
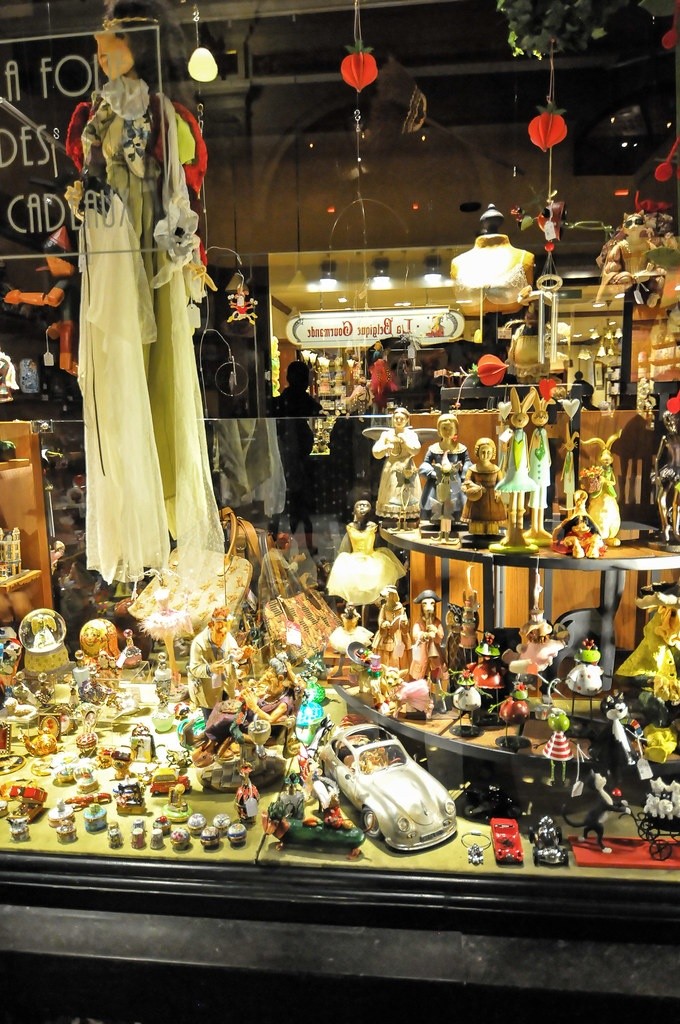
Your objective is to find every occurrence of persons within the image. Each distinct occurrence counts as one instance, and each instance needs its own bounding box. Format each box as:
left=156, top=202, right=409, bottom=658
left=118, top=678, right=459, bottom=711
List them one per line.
left=459, top=438, right=509, bottom=536
left=652, top=408, right=680, bottom=546
left=327, top=499, right=412, bottom=676
left=595, top=208, right=666, bottom=309
left=361, top=407, right=440, bottom=534
left=1, top=519, right=680, bottom=884
left=417, top=414, right=474, bottom=546
left=568, top=370, right=601, bottom=410
left=267, top=359, right=326, bottom=555
left=69, top=0, right=226, bottom=588
left=5, top=225, right=81, bottom=380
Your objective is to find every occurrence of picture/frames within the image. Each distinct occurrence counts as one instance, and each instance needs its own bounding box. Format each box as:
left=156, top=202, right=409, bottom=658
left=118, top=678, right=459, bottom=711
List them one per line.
left=593, top=361, right=604, bottom=387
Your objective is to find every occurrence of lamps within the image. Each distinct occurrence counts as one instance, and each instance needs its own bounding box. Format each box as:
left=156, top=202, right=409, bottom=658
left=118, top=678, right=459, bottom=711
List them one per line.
left=300, top=348, right=356, bottom=386
left=372, top=270, right=390, bottom=282
left=319, top=273, right=337, bottom=284
left=590, top=318, right=623, bottom=357
left=423, top=267, right=442, bottom=279
left=224, top=209, right=249, bottom=293
left=287, top=74, right=310, bottom=289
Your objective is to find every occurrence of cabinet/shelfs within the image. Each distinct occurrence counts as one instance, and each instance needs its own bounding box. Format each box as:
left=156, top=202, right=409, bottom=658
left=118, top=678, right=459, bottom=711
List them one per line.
left=330, top=383, right=680, bottom=777
left=604, top=365, right=620, bottom=401
left=0, top=421, right=53, bottom=626
